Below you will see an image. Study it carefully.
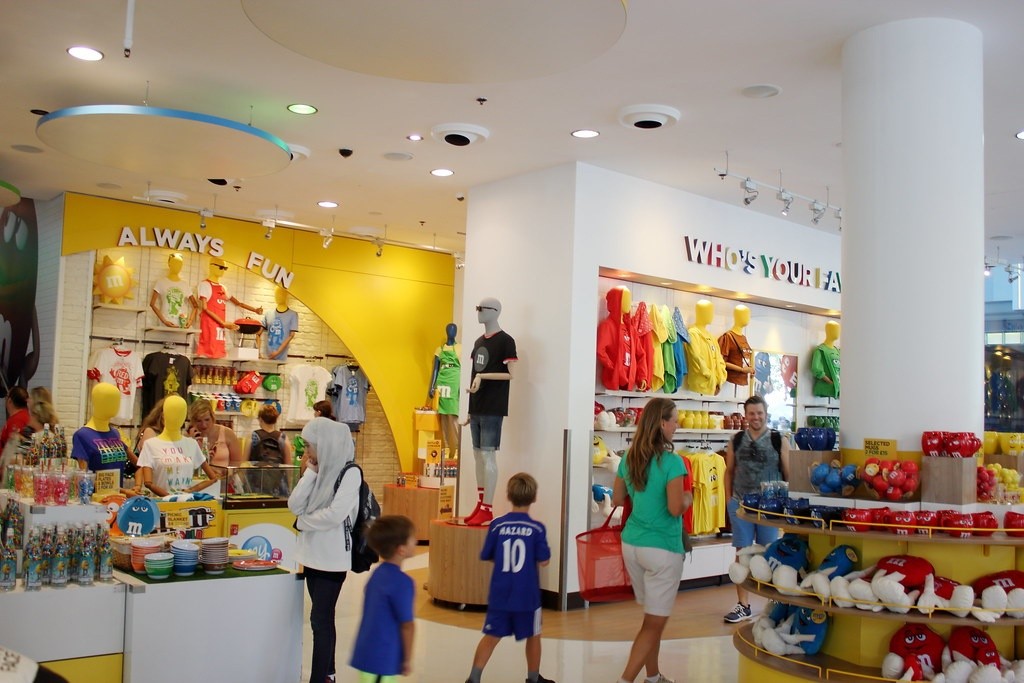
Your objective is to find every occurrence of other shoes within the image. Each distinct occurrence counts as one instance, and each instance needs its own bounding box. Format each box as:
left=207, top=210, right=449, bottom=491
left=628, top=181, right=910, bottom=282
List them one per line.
left=526, top=674, right=556, bottom=683
left=644, top=671, right=675, bottom=683
left=310, top=674, right=336, bottom=683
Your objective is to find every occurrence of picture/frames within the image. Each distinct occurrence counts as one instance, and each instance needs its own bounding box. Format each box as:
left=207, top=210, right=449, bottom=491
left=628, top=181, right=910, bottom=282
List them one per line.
left=750, top=349, right=799, bottom=433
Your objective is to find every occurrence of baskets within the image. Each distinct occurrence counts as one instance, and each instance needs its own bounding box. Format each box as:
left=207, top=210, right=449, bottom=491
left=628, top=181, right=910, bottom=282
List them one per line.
left=111, top=535, right=175, bottom=568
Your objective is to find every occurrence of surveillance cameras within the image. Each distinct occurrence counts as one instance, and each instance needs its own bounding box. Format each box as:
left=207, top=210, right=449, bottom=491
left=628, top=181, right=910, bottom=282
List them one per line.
left=339, top=149, right=353, bottom=158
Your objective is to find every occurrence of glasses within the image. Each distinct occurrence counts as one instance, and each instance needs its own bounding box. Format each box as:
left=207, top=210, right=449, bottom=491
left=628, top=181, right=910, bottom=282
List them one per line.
left=476, top=306, right=498, bottom=312
left=211, top=262, right=229, bottom=270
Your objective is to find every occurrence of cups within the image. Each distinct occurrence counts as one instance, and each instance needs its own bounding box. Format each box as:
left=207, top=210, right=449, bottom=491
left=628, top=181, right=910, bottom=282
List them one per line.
left=6, top=462, right=97, bottom=506
left=948, top=432, right=981, bottom=458
left=1004, top=511, right=1024, bottom=537
left=744, top=493, right=999, bottom=539
left=922, top=431, right=950, bottom=456
left=189, top=391, right=242, bottom=412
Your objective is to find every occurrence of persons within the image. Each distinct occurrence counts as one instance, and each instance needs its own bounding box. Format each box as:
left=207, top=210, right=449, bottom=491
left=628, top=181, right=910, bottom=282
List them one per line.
left=724, top=397, right=792, bottom=623
left=688, top=299, right=729, bottom=396
left=719, top=303, right=755, bottom=386
left=462, top=296, right=521, bottom=526
left=596, top=284, right=648, bottom=393
left=149, top=252, right=198, bottom=328
left=136, top=395, right=217, bottom=495
left=256, top=285, right=300, bottom=360
left=286, top=417, right=361, bottom=683
left=811, top=320, right=842, bottom=400
left=197, top=257, right=263, bottom=359
left=348, top=515, right=417, bottom=683
left=428, top=322, right=462, bottom=459
left=70, top=384, right=143, bottom=498
left=239, top=404, right=294, bottom=496
left=187, top=395, right=240, bottom=494
left=313, top=399, right=336, bottom=422
left=0, top=386, right=60, bottom=488
left=611, top=397, right=694, bottom=683
left=135, top=391, right=178, bottom=454
left=464, top=472, right=556, bottom=683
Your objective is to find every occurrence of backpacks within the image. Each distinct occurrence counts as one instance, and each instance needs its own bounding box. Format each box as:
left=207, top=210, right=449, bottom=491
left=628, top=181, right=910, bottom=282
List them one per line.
left=247, top=430, right=285, bottom=490
left=334, top=463, right=381, bottom=573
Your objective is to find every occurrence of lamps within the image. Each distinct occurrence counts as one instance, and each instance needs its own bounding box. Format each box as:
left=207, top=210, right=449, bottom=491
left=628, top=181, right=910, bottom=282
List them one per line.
left=321, top=233, right=333, bottom=249
left=371, top=240, right=384, bottom=257
left=198, top=210, right=214, bottom=230
left=1004, top=267, right=1020, bottom=284
left=776, top=192, right=794, bottom=216
left=833, top=211, right=844, bottom=231
left=739, top=180, right=759, bottom=205
left=808, top=203, right=826, bottom=224
left=261, top=221, right=276, bottom=240
left=452, top=251, right=465, bottom=272
left=984, top=258, right=996, bottom=276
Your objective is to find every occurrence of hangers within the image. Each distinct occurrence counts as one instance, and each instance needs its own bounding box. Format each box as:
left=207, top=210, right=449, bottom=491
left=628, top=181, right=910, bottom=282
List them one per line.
left=304, top=355, right=358, bottom=369
left=110, top=336, right=178, bottom=355
left=682, top=438, right=714, bottom=456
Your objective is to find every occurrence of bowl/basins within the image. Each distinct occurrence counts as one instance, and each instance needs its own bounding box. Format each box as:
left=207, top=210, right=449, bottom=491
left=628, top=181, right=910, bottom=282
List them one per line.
left=130, top=538, right=229, bottom=580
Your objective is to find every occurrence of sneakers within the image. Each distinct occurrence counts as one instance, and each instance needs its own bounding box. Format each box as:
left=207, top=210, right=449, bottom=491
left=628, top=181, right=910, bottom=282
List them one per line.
left=723, top=601, right=752, bottom=622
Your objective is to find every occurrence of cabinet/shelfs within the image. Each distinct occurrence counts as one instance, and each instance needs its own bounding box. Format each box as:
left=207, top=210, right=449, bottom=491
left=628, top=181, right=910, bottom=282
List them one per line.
left=595, top=390, right=746, bottom=469
left=732, top=501, right=1024, bottom=683
left=92, top=302, right=289, bottom=414
left=805, top=404, right=839, bottom=436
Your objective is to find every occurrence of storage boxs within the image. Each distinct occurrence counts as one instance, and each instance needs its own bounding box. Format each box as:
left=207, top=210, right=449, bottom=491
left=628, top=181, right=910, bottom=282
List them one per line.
left=229, top=347, right=259, bottom=360
left=984, top=450, right=1024, bottom=487
left=788, top=450, right=841, bottom=492
left=209, top=460, right=302, bottom=510
left=921, top=456, right=978, bottom=505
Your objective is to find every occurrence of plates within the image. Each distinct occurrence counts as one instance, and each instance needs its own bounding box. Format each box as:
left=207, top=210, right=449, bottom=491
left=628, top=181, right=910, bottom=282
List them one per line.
left=227, top=549, right=275, bottom=570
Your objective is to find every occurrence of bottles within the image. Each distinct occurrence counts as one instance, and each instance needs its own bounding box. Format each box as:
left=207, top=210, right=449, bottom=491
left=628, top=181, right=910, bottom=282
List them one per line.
left=61, top=424, right=67, bottom=458
left=40, top=423, right=51, bottom=458
left=0, top=500, right=113, bottom=590
left=426, top=463, right=457, bottom=477
left=676, top=409, right=750, bottom=430
left=51, top=424, right=62, bottom=458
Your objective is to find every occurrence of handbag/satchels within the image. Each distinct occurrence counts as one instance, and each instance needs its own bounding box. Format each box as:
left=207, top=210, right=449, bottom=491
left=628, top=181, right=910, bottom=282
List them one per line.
left=575, top=494, right=636, bottom=602
left=122, top=452, right=141, bottom=478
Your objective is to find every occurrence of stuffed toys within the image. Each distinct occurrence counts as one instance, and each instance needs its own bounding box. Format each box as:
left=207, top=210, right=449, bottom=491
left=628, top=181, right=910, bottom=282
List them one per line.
left=727, top=529, right=1024, bottom=683
